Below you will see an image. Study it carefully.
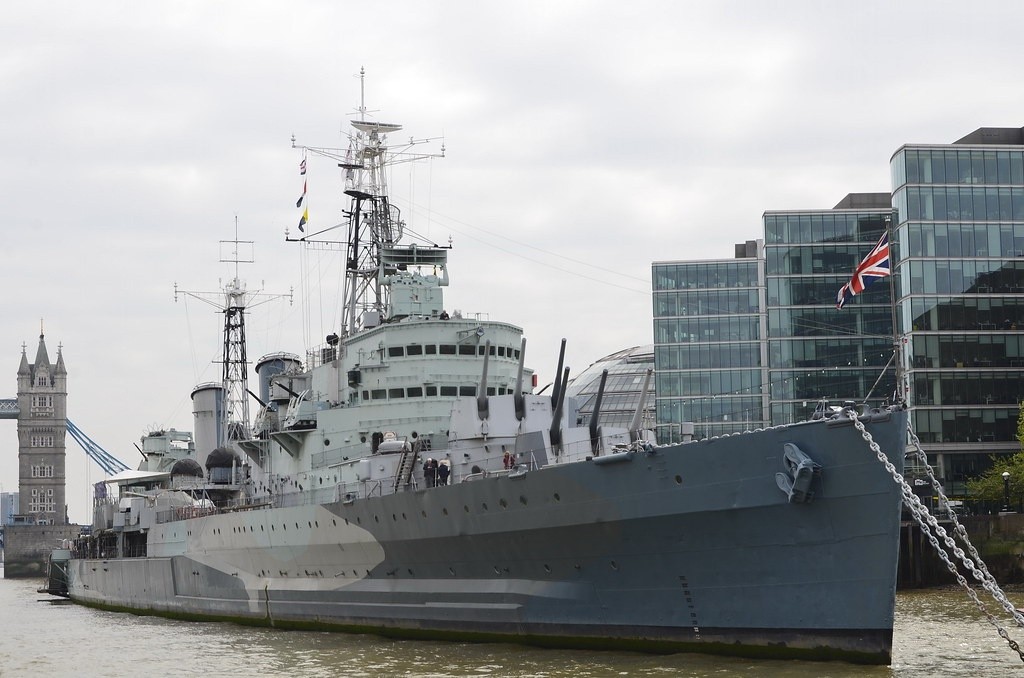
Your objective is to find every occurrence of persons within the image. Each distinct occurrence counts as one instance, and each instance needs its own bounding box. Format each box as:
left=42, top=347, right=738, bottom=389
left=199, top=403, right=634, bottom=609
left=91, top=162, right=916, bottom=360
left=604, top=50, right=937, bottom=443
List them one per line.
left=439, top=310, right=449, bottom=320
left=423, top=451, right=515, bottom=488
left=379, top=315, right=387, bottom=325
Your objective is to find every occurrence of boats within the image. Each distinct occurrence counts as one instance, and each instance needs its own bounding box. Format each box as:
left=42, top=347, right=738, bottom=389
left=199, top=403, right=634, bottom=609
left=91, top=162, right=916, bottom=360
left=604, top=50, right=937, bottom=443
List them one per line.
left=37, top=64, right=910, bottom=667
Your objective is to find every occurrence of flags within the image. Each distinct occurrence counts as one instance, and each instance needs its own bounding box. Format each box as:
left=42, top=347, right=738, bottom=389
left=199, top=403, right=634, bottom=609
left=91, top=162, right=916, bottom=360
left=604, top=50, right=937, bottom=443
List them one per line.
left=344, top=144, right=353, bottom=164
left=297, top=159, right=308, bottom=232
left=836, top=230, right=890, bottom=310
